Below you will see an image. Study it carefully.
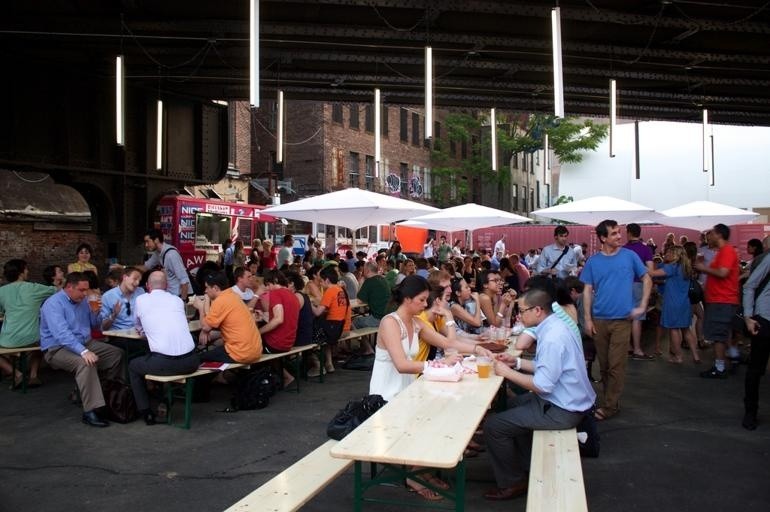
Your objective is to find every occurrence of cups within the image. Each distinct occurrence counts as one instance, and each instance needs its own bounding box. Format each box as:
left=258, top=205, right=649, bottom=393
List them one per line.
left=442, top=346, right=462, bottom=363
left=87, top=292, right=102, bottom=312
left=479, top=325, right=509, bottom=350
left=475, top=354, right=494, bottom=381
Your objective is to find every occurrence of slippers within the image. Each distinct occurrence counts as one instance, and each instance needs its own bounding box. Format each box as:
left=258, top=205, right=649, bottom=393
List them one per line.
left=405, top=471, right=449, bottom=500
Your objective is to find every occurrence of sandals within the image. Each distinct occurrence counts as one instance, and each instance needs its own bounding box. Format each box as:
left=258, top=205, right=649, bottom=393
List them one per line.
left=594, top=408, right=608, bottom=421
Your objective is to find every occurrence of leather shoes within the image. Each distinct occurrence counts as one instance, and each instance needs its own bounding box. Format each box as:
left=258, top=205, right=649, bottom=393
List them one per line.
left=80, top=409, right=109, bottom=427
left=485, top=481, right=526, bottom=500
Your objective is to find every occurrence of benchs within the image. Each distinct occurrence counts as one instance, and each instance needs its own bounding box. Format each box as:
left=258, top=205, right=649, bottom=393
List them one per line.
left=101, top=298, right=382, bottom=427
left=526, top=428, right=588, bottom=511
left=1, top=344, right=43, bottom=392
left=221, top=438, right=350, bottom=511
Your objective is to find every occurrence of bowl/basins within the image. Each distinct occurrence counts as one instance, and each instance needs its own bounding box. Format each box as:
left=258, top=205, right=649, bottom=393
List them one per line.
left=187, top=295, right=206, bottom=304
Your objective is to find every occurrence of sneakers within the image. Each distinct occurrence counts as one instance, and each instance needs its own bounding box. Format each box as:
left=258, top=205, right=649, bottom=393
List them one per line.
left=700, top=367, right=728, bottom=378
left=278, top=364, right=336, bottom=391
left=143, top=409, right=156, bottom=425
left=628, top=340, right=706, bottom=364
left=9, top=374, right=24, bottom=390
left=742, top=409, right=760, bottom=431
left=465, top=442, right=486, bottom=457
left=28, top=377, right=42, bottom=385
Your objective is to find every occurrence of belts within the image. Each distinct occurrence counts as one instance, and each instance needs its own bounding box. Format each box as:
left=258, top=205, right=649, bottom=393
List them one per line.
left=151, top=351, right=193, bottom=359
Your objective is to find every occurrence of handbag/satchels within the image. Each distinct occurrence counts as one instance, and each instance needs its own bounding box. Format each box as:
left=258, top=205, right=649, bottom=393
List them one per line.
left=326, top=393, right=388, bottom=440
left=341, top=350, right=374, bottom=371
left=93, top=378, right=140, bottom=424
left=321, top=318, right=345, bottom=346
left=186, top=269, right=203, bottom=296
left=688, top=275, right=704, bottom=305
left=231, top=363, right=274, bottom=411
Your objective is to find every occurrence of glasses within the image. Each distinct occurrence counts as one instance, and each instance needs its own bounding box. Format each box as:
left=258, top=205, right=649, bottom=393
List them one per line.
left=520, top=306, right=535, bottom=313
left=125, top=301, right=131, bottom=316
left=488, top=278, right=498, bottom=283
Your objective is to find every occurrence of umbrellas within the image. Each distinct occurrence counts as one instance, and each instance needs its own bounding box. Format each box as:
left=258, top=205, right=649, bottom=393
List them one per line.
left=260, top=186, right=441, bottom=256
left=530, top=194, right=679, bottom=228
left=654, top=200, right=762, bottom=233
left=395, top=202, right=534, bottom=252
